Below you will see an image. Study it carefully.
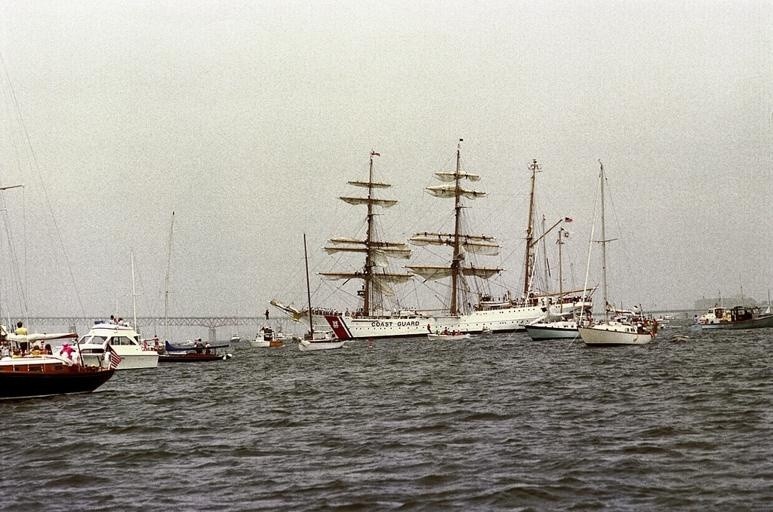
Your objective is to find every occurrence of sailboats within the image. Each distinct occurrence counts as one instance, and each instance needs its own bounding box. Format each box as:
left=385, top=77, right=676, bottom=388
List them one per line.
left=266, top=139, right=640, bottom=340
left=575, top=158, right=658, bottom=346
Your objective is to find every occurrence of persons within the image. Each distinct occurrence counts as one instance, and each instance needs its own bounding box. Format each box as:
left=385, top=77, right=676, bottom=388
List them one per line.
left=344, top=307, right=363, bottom=319
left=140, top=333, right=162, bottom=350
left=193, top=338, right=213, bottom=356
left=634, top=315, right=658, bottom=339
left=324, top=332, right=339, bottom=342
left=14, top=320, right=76, bottom=362
left=107, top=315, right=130, bottom=328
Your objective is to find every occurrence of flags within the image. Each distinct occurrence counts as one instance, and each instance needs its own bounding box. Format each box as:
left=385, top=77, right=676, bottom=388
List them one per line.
left=109, top=349, right=121, bottom=370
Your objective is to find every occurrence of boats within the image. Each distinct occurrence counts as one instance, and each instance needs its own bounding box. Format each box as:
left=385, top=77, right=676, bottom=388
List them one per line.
left=230, top=330, right=241, bottom=342
left=0, top=210, right=126, bottom=402
left=157, top=339, right=232, bottom=363
left=696, top=283, right=773, bottom=331
left=296, top=231, right=347, bottom=352
left=51, top=314, right=161, bottom=372
left=425, top=332, right=473, bottom=342
left=246, top=308, right=284, bottom=348
